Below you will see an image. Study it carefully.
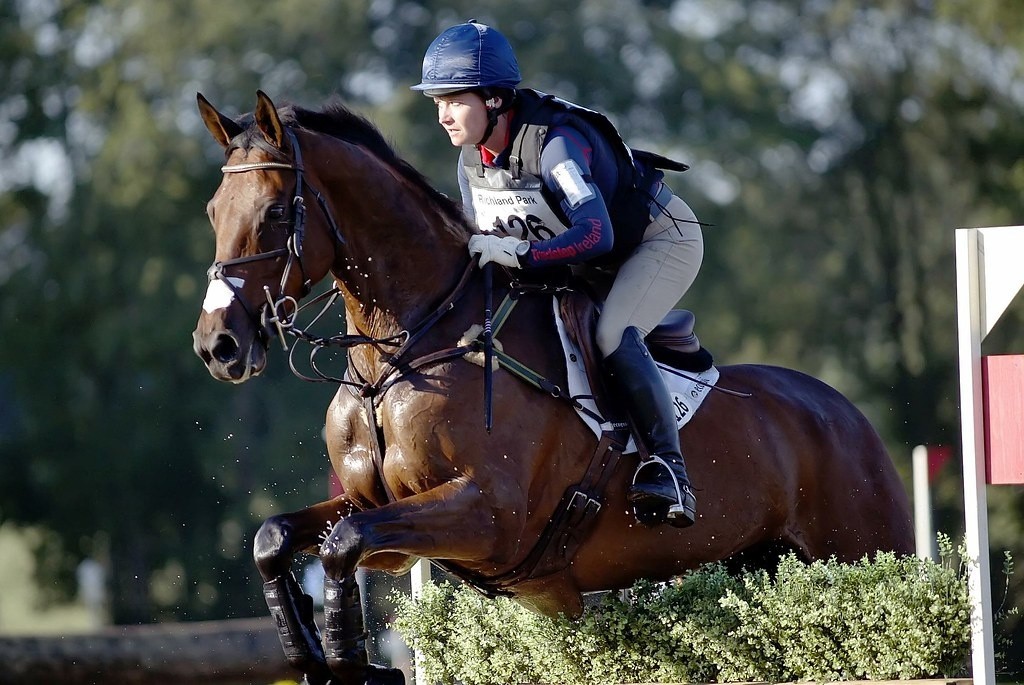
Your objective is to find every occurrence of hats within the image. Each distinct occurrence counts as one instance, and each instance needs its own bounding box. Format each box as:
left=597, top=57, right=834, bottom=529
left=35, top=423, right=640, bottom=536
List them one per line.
left=410, top=19, right=523, bottom=96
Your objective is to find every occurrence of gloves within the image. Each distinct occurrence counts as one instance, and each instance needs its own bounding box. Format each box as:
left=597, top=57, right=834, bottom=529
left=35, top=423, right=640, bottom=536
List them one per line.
left=468, top=234, right=530, bottom=270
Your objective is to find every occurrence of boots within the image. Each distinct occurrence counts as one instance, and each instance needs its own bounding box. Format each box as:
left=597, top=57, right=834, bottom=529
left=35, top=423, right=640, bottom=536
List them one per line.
left=599, top=326, right=696, bottom=527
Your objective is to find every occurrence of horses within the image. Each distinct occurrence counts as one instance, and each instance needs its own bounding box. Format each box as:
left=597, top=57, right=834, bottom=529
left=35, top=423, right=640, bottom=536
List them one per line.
left=193, top=90, right=914, bottom=685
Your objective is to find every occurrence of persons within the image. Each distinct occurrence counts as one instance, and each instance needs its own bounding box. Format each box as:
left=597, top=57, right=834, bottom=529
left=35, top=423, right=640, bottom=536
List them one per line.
left=407, top=16, right=704, bottom=527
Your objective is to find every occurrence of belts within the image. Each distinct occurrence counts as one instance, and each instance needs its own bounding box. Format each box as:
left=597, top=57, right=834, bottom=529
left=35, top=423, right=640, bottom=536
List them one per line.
left=647, top=181, right=672, bottom=230
